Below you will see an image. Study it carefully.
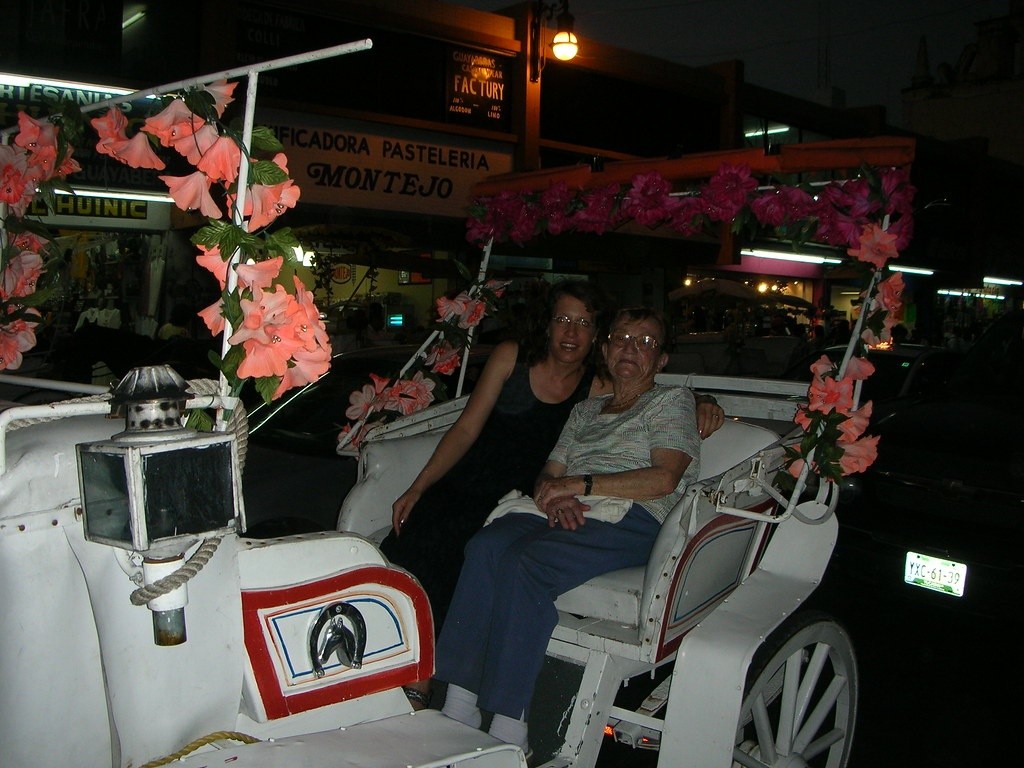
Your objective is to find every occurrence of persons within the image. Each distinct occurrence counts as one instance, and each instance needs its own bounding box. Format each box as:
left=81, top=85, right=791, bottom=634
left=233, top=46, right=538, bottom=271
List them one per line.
left=378, top=280, right=725, bottom=713
left=430, top=305, right=701, bottom=759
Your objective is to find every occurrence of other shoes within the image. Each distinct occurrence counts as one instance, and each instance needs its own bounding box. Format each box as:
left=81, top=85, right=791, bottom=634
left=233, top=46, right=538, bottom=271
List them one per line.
left=525, top=747, right=534, bottom=764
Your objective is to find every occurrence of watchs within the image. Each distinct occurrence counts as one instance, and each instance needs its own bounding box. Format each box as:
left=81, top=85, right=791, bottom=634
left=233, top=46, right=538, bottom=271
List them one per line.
left=584, top=474, right=593, bottom=496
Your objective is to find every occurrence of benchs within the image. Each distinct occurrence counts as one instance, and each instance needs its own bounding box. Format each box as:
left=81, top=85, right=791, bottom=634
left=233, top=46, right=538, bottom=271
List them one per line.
left=336, top=419, right=787, bottom=663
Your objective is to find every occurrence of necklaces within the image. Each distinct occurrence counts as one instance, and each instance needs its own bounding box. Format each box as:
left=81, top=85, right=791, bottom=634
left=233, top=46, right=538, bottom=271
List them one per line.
left=608, top=390, right=650, bottom=406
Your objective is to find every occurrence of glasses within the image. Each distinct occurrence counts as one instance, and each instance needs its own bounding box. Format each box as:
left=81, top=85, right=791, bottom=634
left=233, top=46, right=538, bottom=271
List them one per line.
left=607, top=332, right=661, bottom=350
left=552, top=316, right=596, bottom=327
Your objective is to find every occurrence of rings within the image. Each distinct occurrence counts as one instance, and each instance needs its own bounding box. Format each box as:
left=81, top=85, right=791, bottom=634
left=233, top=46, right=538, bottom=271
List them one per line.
left=557, top=508, right=564, bottom=514
left=712, top=414, right=719, bottom=419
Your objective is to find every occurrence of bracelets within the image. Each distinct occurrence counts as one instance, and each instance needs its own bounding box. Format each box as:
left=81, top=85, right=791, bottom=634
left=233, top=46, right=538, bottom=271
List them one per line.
left=695, top=395, right=718, bottom=407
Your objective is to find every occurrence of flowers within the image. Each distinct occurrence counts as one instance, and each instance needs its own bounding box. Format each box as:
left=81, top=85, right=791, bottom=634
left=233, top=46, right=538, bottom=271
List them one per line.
left=0, top=77, right=333, bottom=432
left=332, top=165, right=917, bottom=493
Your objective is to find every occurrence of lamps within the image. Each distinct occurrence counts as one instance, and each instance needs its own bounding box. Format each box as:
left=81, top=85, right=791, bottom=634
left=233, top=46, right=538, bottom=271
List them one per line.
left=529, top=0, right=579, bottom=83
